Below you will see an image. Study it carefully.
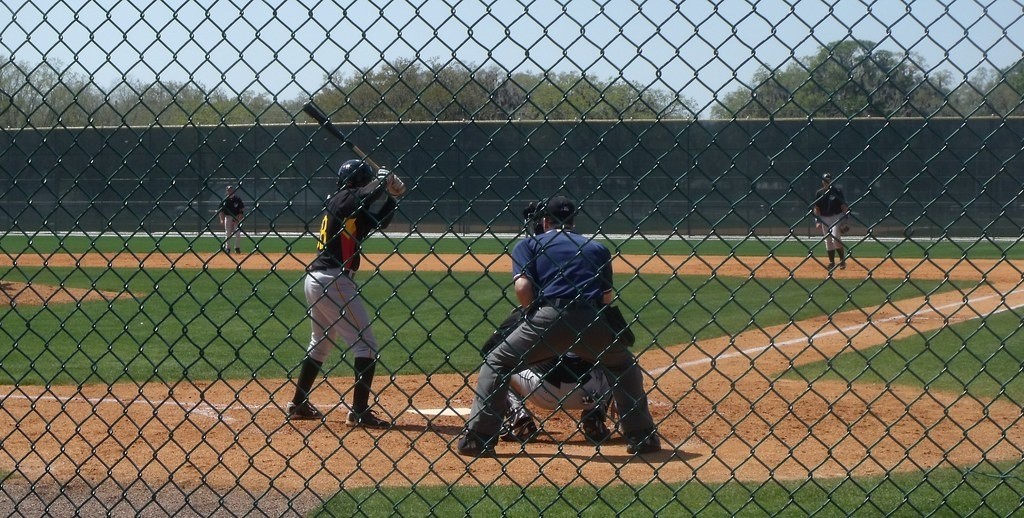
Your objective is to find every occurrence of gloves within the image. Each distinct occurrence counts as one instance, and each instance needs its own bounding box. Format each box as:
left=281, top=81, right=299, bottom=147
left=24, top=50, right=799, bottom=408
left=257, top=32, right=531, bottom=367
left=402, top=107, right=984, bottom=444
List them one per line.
left=376, top=166, right=390, bottom=183
left=389, top=175, right=405, bottom=196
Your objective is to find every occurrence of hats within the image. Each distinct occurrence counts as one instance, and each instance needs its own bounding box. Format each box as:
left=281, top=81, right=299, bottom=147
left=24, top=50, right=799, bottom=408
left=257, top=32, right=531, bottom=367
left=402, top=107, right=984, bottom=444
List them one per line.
left=523, top=200, right=544, bottom=223
left=226, top=186, right=233, bottom=191
left=544, top=195, right=574, bottom=215
left=822, top=173, right=832, bottom=181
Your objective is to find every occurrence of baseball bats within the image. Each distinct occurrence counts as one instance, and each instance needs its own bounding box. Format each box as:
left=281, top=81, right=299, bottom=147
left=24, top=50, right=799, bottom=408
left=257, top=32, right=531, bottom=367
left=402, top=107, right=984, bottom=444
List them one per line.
left=301, top=100, right=407, bottom=196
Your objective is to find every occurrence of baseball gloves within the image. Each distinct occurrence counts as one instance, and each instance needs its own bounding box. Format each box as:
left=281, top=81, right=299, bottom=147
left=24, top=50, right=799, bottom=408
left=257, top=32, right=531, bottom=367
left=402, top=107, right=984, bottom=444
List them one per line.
left=840, top=220, right=849, bottom=234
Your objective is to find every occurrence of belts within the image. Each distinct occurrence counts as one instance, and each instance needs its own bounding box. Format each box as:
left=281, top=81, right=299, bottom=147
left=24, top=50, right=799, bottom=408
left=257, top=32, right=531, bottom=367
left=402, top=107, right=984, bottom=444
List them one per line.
left=315, top=266, right=355, bottom=279
left=540, top=298, right=596, bottom=309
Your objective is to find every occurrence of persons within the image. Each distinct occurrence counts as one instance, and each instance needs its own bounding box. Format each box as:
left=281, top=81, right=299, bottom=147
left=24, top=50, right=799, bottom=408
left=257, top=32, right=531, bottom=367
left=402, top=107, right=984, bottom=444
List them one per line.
left=219, top=186, right=245, bottom=254
left=814, top=174, right=849, bottom=270
left=459, top=197, right=660, bottom=455
left=285, top=161, right=406, bottom=427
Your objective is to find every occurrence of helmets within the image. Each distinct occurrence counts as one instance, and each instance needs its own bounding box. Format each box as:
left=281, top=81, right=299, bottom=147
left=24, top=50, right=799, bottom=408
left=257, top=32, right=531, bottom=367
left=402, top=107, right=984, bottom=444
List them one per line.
left=337, top=159, right=373, bottom=186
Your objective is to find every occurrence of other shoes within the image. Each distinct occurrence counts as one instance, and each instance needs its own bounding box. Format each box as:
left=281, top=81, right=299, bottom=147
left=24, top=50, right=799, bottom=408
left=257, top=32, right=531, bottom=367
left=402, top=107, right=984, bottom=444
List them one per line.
left=840, top=262, right=846, bottom=270
left=225, top=249, right=230, bottom=254
left=827, top=263, right=835, bottom=269
left=235, top=249, right=241, bottom=254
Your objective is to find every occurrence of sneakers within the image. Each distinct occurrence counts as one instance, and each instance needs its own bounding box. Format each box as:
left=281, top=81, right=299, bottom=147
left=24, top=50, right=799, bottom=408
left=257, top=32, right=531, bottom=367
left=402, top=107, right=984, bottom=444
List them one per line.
left=626, top=432, right=660, bottom=453
left=458, top=435, right=495, bottom=455
left=502, top=421, right=537, bottom=442
left=346, top=409, right=390, bottom=429
left=285, top=402, right=322, bottom=420
left=583, top=418, right=611, bottom=441
